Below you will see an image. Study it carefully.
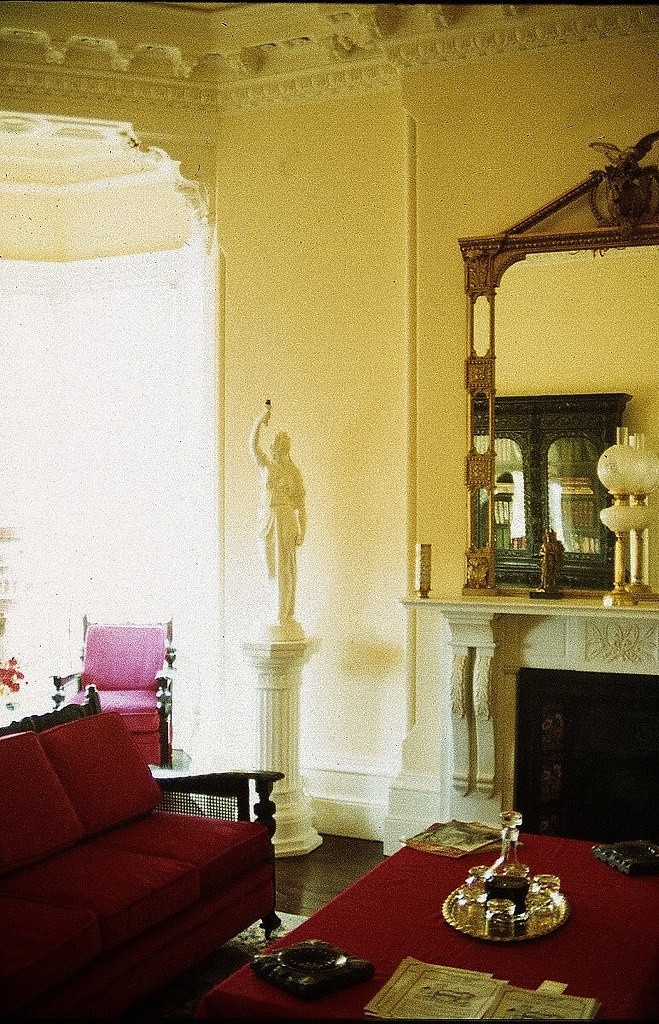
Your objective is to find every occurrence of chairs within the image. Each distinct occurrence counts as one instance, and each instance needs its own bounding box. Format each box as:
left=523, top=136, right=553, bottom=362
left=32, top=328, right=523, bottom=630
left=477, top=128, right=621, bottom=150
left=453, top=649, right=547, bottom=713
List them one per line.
left=51, top=618, right=177, bottom=768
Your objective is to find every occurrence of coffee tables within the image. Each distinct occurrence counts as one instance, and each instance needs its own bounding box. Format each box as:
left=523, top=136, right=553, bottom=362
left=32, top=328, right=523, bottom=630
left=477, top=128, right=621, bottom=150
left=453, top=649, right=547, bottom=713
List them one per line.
left=196, top=824, right=659, bottom=1023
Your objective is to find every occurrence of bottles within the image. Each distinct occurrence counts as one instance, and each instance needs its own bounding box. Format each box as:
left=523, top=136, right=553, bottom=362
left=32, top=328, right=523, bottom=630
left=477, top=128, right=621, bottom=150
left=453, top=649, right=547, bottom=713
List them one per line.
left=484, top=811, right=529, bottom=921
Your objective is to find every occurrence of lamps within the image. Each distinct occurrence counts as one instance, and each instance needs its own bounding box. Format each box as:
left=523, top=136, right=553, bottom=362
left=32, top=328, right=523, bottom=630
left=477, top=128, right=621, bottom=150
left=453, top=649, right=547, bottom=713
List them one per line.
left=599, top=427, right=654, bottom=607
left=622, top=431, right=659, bottom=603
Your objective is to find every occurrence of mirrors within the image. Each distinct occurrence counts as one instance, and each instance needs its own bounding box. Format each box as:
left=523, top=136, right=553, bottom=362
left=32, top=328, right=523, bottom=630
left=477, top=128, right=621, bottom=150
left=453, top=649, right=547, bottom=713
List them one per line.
left=453, top=129, right=659, bottom=602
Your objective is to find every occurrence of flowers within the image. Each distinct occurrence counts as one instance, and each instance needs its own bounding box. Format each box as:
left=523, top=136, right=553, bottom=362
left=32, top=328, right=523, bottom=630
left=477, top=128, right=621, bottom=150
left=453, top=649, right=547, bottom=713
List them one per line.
left=0, top=657, right=24, bottom=696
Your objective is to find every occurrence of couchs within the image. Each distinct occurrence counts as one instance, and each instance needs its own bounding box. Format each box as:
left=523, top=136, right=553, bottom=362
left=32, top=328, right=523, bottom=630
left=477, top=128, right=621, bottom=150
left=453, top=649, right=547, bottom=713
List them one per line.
left=0, top=697, right=286, bottom=1024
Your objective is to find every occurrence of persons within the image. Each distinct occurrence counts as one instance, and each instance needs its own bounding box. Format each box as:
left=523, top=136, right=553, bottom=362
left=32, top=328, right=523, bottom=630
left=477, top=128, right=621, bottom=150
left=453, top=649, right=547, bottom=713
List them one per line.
left=248, top=412, right=306, bottom=625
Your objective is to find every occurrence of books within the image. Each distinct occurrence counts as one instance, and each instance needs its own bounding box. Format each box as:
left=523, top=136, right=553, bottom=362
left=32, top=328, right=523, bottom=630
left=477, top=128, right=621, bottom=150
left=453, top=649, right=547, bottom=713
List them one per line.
left=547, top=437, right=601, bottom=554
left=473, top=435, right=525, bottom=550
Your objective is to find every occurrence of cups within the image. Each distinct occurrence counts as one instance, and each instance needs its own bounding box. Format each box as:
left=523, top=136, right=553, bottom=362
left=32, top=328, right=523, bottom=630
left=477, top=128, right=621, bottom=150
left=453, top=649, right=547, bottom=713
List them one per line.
left=464, top=866, right=489, bottom=902
left=485, top=900, right=515, bottom=938
left=530, top=874, right=561, bottom=915
